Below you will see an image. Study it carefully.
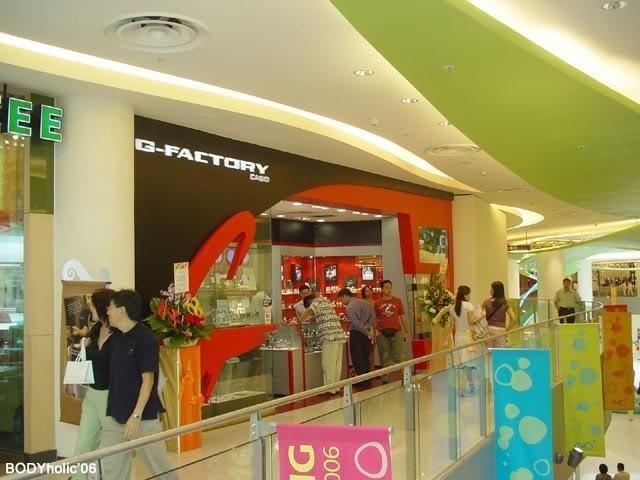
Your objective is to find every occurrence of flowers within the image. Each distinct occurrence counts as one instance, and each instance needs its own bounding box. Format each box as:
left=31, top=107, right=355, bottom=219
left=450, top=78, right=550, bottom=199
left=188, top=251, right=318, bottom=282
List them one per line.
left=423, top=270, right=452, bottom=328
left=142, top=283, right=215, bottom=350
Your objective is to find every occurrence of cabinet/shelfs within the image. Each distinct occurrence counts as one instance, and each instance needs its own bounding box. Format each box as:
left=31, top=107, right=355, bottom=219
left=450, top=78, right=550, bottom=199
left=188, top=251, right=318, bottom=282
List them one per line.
left=281, top=254, right=383, bottom=322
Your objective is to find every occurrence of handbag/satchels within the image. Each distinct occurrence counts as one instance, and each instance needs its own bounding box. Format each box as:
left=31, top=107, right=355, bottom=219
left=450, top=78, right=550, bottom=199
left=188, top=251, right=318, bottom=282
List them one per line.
left=471, top=320, right=490, bottom=344
left=378, top=327, right=401, bottom=340
left=62, top=337, right=96, bottom=385
left=63, top=385, right=86, bottom=400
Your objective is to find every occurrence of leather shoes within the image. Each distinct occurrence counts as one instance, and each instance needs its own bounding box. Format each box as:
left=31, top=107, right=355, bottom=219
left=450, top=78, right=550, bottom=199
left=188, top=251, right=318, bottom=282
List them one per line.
left=326, top=388, right=341, bottom=396
left=352, top=379, right=390, bottom=387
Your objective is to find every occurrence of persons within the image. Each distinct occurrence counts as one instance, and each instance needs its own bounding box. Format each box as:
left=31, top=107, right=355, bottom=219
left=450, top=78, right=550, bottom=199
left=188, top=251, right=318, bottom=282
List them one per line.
left=68, top=287, right=117, bottom=480
left=338, top=288, right=376, bottom=387
left=480, top=281, right=516, bottom=349
left=552, top=278, right=585, bottom=323
left=595, top=463, right=612, bottom=480
left=442, top=284, right=484, bottom=398
left=360, top=285, right=376, bottom=359
left=99, top=287, right=179, bottom=480
left=572, top=281, right=578, bottom=290
left=292, top=285, right=312, bottom=317
left=613, top=462, right=630, bottom=480
left=371, top=279, right=408, bottom=384
left=593, top=278, right=634, bottom=297
left=298, top=293, right=344, bottom=396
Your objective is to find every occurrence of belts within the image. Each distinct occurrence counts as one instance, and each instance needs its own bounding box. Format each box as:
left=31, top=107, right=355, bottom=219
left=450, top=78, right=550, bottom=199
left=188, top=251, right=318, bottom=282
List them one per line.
left=559, top=307, right=574, bottom=310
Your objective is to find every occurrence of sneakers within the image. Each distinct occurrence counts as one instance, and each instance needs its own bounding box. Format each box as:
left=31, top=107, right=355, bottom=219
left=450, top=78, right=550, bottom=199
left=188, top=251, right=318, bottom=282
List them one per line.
left=457, top=384, right=478, bottom=398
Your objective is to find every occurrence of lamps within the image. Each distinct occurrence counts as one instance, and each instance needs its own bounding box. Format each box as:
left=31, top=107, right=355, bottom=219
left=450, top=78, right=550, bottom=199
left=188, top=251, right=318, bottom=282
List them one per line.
left=567, top=448, right=583, bottom=468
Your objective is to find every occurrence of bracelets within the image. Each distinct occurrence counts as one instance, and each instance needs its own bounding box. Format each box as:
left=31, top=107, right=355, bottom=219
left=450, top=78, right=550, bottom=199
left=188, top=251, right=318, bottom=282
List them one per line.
left=130, top=415, right=141, bottom=419
